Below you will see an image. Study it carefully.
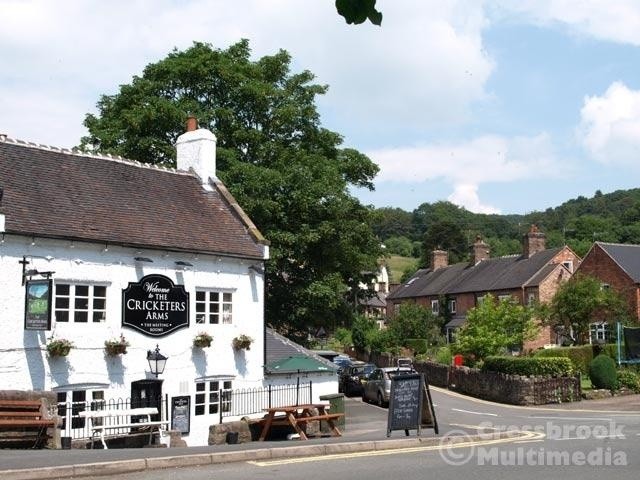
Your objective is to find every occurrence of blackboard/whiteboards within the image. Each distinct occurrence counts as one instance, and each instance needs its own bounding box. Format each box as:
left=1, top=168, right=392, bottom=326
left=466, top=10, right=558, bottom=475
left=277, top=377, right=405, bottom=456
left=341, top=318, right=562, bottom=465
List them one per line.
left=388, top=373, right=424, bottom=430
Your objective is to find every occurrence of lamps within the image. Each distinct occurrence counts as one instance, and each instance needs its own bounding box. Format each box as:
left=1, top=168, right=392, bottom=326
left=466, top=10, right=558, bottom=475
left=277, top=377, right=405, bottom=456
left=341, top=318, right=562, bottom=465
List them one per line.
left=146, top=344, right=168, bottom=377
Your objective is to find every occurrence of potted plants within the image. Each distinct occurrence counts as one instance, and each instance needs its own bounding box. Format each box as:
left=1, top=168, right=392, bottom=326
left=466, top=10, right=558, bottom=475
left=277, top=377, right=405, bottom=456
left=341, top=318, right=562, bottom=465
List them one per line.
left=47, top=339, right=71, bottom=357
left=104, top=332, right=128, bottom=356
left=194, top=328, right=212, bottom=348
left=233, top=334, right=256, bottom=352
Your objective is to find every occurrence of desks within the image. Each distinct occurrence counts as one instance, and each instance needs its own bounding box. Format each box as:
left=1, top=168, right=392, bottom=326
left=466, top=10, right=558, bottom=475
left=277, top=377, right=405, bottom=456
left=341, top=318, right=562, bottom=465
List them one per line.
left=259, top=404, right=341, bottom=441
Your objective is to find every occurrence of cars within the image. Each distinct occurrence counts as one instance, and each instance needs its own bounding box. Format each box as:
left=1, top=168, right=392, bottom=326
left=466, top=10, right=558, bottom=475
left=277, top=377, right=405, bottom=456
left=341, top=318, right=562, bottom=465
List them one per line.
left=333, top=356, right=418, bottom=409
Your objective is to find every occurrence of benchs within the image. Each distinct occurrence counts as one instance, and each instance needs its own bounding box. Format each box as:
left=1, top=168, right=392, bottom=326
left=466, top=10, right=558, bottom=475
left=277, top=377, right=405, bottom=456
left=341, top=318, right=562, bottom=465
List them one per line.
left=297, top=413, right=345, bottom=424
left=79, top=407, right=170, bottom=450
left=0, top=400, right=55, bottom=449
left=250, top=413, right=302, bottom=425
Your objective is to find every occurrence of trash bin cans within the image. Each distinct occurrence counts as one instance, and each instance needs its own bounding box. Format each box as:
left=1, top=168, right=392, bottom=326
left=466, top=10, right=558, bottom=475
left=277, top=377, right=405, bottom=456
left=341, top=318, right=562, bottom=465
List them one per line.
left=320, top=393, right=345, bottom=437
